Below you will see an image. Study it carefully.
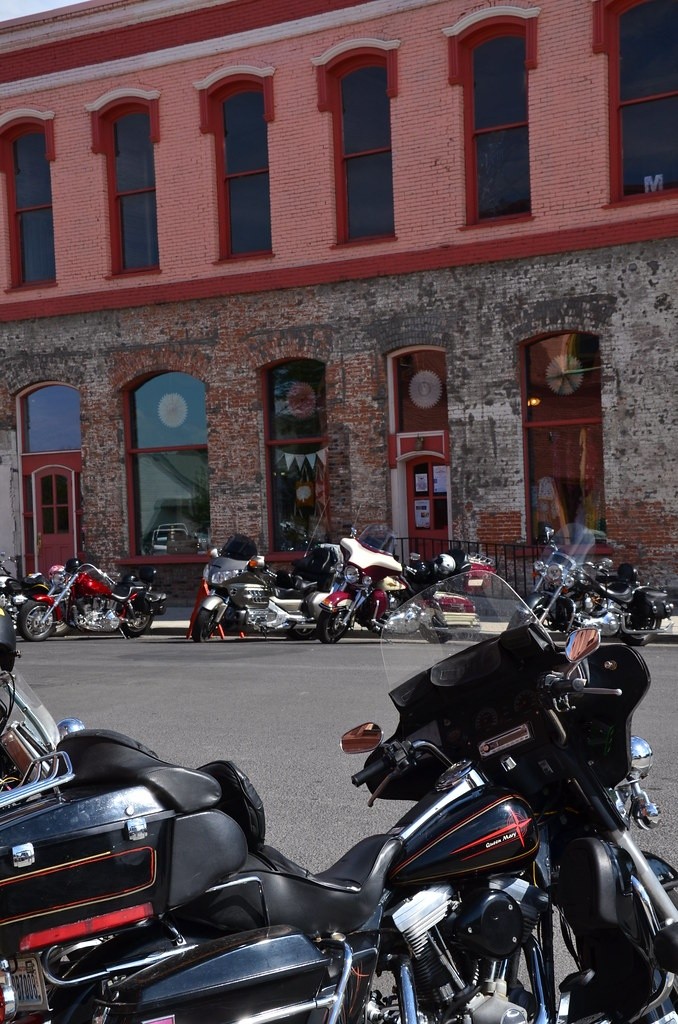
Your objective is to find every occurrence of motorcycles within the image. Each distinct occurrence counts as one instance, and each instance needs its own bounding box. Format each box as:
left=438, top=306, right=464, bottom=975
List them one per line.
left=0, top=567, right=678, bottom=1024
left=0, top=601, right=86, bottom=790
left=0, top=520, right=500, bottom=648
left=504, top=523, right=674, bottom=646
